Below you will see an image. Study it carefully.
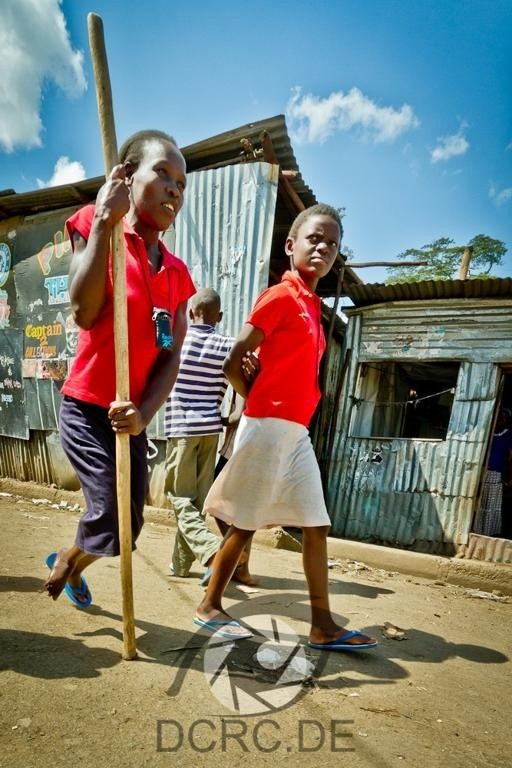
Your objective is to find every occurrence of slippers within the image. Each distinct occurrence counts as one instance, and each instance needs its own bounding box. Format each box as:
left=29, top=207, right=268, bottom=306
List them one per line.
left=308, top=629, right=378, bottom=649
left=199, top=568, right=257, bottom=586
left=194, top=617, right=252, bottom=639
left=46, top=553, right=91, bottom=609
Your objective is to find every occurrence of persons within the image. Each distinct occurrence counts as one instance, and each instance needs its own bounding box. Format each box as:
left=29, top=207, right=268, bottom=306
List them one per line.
left=44, top=130, right=198, bottom=601
left=193, top=205, right=379, bottom=651
left=157, top=289, right=241, bottom=587
left=214, top=352, right=260, bottom=590
